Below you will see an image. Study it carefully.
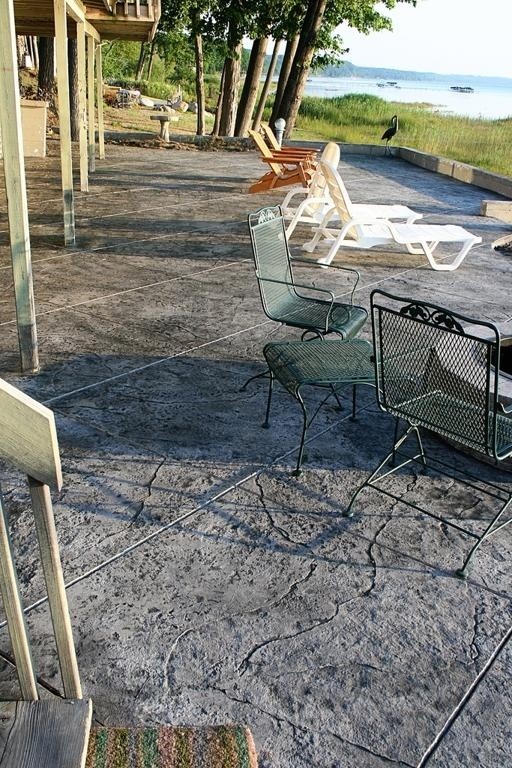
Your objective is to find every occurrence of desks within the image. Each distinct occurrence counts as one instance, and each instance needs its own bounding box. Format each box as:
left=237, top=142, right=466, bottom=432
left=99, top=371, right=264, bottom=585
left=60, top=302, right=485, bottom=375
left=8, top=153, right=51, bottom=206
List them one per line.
left=150, top=116, right=178, bottom=142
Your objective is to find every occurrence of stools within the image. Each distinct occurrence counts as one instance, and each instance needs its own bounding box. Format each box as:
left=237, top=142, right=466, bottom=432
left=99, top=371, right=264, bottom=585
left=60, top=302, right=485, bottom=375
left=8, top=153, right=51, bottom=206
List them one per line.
left=263, top=339, right=417, bottom=476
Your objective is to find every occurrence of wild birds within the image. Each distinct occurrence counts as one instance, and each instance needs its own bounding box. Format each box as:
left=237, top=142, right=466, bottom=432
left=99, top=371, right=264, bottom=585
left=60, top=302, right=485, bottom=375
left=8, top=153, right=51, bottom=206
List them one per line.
left=381, top=115, right=398, bottom=157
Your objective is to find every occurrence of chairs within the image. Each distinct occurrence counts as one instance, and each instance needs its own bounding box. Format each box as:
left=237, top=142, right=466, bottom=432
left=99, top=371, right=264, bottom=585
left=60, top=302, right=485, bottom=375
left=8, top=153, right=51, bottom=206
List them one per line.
left=343, top=289, right=512, bottom=578
left=248, top=205, right=368, bottom=410
left=248, top=123, right=483, bottom=271
